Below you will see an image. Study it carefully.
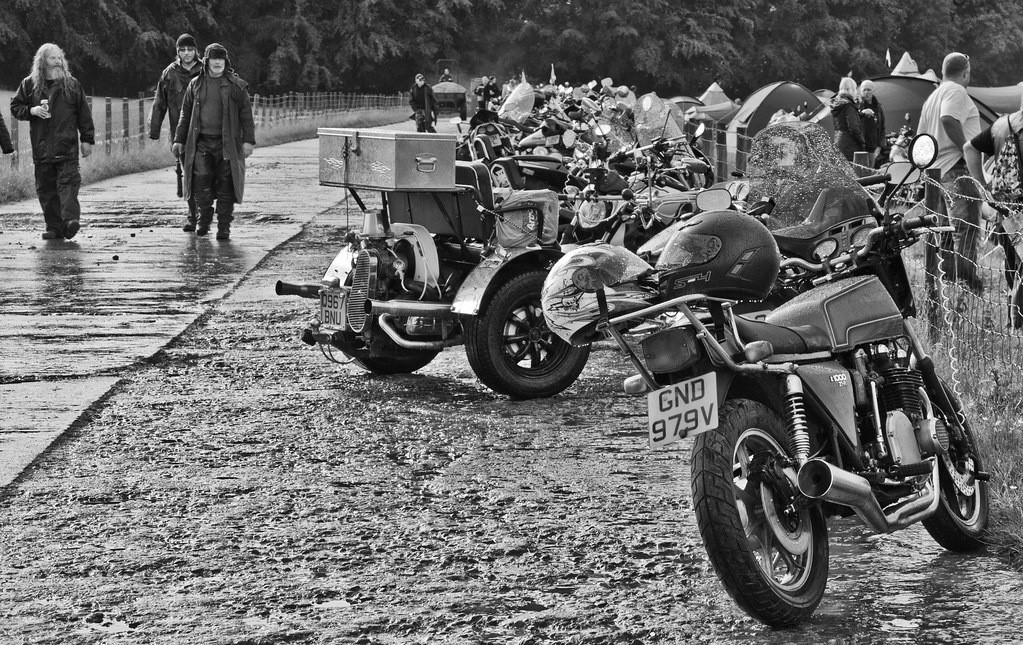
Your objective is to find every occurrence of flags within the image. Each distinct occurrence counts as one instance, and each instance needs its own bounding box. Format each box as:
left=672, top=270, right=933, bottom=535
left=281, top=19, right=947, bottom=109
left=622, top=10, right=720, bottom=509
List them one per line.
left=886, top=49, right=892, bottom=67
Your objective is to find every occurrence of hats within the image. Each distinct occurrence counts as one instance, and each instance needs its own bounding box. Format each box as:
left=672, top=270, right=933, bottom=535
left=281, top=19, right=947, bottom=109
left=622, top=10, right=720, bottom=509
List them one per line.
left=414, top=73, right=424, bottom=81
left=202, top=43, right=230, bottom=77
left=176, top=33, right=200, bottom=63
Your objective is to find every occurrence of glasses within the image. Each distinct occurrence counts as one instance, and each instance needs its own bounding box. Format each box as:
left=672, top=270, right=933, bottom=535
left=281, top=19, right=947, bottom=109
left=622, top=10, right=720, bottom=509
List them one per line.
left=963, top=53, right=969, bottom=60
left=178, top=47, right=193, bottom=52
left=419, top=76, right=424, bottom=81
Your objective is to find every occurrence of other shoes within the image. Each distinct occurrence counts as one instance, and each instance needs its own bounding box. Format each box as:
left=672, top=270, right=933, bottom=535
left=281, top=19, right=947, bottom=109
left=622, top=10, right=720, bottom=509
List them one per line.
left=217, top=228, right=230, bottom=240
left=65, top=219, right=80, bottom=239
left=42, top=229, right=64, bottom=239
left=183, top=220, right=196, bottom=231
left=197, top=226, right=208, bottom=235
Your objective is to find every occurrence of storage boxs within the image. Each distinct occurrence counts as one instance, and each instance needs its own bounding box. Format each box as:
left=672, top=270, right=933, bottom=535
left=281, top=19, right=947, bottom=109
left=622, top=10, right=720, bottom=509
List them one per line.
left=315, top=126, right=457, bottom=192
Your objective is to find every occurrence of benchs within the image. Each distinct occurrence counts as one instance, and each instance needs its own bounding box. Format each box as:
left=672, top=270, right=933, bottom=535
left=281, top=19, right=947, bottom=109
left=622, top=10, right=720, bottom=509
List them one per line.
left=382, top=134, right=559, bottom=262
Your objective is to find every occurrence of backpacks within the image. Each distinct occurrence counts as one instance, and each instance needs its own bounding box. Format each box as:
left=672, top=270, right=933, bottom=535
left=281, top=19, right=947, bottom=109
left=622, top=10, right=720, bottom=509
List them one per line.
left=991, top=112, right=1023, bottom=214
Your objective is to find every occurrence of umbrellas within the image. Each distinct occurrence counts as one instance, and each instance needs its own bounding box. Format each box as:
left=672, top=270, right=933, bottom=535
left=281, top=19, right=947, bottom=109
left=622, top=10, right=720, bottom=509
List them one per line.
left=176, top=160, right=183, bottom=197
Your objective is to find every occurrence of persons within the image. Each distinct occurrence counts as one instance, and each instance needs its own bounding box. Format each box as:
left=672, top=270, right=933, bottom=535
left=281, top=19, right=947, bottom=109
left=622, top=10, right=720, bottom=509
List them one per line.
left=11, top=43, right=95, bottom=240
left=474, top=76, right=488, bottom=110
left=830, top=77, right=865, bottom=161
left=172, top=42, right=256, bottom=241
left=0, top=109, right=18, bottom=157
left=408, top=74, right=439, bottom=133
left=962, top=82, right=1023, bottom=332
left=483, top=76, right=501, bottom=110
left=148, top=33, right=204, bottom=164
left=854, top=80, right=886, bottom=170
left=913, top=52, right=983, bottom=296
left=440, top=69, right=453, bottom=82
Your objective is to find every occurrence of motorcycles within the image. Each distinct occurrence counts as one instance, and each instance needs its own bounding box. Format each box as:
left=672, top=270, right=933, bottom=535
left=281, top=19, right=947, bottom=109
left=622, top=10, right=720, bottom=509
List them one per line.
left=457, top=69, right=686, bottom=264
left=310, top=121, right=738, bottom=402
left=543, top=128, right=995, bottom=627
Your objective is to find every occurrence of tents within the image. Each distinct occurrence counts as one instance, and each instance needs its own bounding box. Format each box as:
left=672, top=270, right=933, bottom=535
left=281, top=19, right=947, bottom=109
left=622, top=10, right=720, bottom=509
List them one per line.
left=670, top=51, right=1000, bottom=202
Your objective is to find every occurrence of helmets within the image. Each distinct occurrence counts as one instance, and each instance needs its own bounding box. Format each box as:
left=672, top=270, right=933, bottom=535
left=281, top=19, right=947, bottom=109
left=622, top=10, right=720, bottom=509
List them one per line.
left=654, top=210, right=781, bottom=301
left=540, top=241, right=662, bottom=346
